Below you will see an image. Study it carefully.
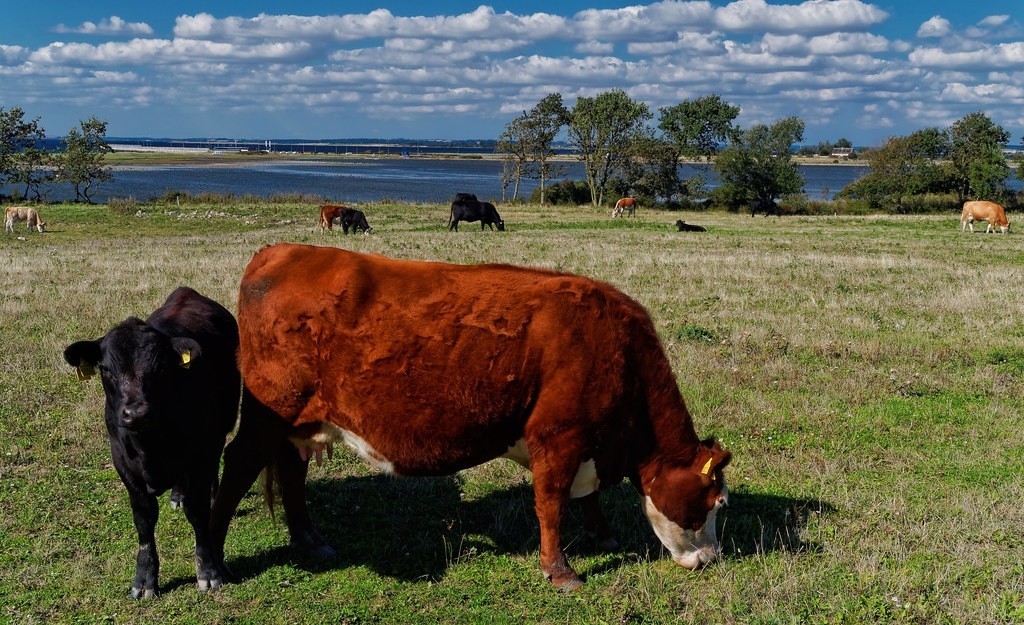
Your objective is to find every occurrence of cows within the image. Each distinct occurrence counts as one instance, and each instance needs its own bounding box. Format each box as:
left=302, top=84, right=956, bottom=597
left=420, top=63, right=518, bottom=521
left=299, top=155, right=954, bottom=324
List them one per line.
left=318, top=205, right=373, bottom=238
left=1, top=207, right=47, bottom=235
left=62, top=286, right=327, bottom=601
left=445, top=193, right=504, bottom=238
left=960, top=200, right=1011, bottom=234
left=664, top=197, right=784, bottom=233
left=610, top=197, right=644, bottom=219
left=212, top=242, right=731, bottom=591
